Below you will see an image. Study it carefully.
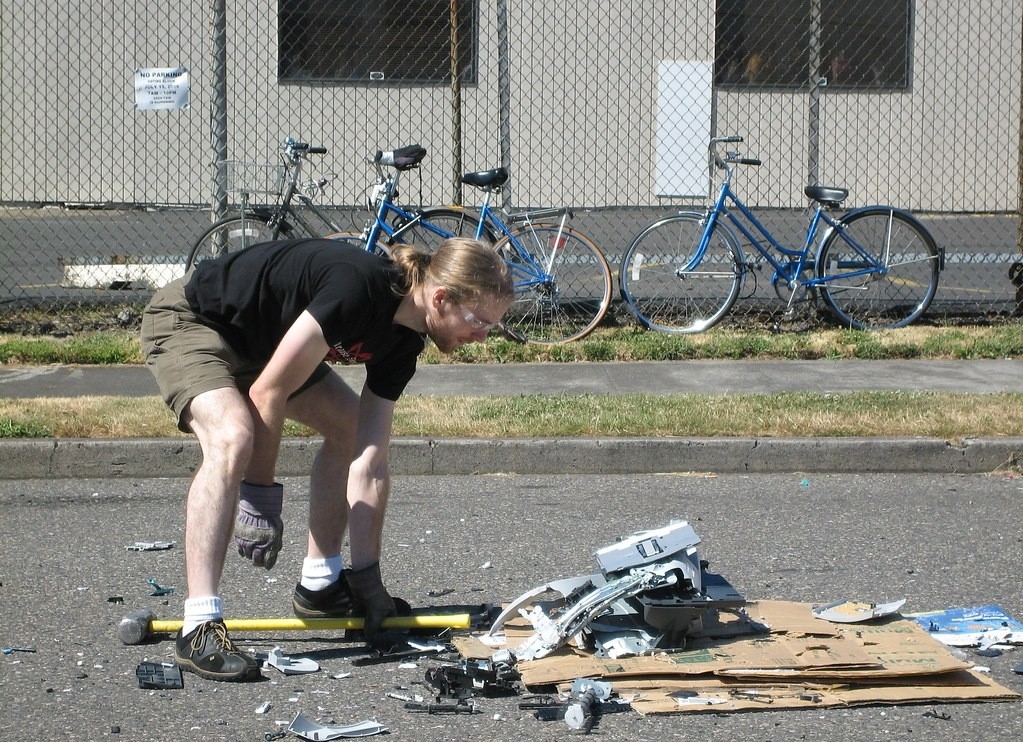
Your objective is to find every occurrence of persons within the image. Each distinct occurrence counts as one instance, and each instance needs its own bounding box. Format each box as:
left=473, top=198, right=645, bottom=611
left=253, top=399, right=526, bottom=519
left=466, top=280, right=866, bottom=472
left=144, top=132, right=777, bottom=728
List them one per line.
left=717, top=39, right=897, bottom=87
left=141, top=236, right=514, bottom=682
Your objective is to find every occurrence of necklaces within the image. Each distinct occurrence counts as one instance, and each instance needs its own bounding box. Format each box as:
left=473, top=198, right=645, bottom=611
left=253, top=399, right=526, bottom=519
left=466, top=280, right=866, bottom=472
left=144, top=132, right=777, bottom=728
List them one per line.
left=416, top=326, right=430, bottom=344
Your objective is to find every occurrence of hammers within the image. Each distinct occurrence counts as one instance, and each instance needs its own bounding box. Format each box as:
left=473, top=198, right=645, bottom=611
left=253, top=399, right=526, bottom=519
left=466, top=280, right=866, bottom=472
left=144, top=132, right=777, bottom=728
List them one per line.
left=118, top=609, right=469, bottom=644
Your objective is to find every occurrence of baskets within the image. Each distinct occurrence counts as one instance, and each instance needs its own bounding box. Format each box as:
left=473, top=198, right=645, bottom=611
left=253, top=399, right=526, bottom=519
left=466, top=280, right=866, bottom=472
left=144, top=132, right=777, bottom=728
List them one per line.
left=217, top=157, right=290, bottom=218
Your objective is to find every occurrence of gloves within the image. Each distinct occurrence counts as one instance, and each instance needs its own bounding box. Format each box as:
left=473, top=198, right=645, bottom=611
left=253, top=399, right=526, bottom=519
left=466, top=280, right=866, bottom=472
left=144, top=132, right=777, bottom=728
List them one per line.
left=343, top=561, right=398, bottom=652
left=233, top=477, right=284, bottom=570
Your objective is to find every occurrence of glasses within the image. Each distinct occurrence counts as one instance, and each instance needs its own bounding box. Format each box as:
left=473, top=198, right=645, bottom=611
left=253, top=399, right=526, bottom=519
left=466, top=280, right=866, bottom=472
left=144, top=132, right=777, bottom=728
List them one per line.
left=450, top=297, right=499, bottom=330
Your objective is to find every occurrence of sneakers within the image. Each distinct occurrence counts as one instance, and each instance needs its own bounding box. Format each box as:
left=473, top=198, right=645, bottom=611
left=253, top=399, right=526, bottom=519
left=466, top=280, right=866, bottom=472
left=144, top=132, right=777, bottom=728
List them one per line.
left=292, top=567, right=411, bottom=618
left=173, top=617, right=262, bottom=682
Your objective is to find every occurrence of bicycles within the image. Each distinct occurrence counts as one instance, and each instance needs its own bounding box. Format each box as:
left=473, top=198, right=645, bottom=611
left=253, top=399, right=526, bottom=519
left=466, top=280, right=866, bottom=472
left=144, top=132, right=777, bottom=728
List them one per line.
left=316, top=145, right=613, bottom=348
left=183, top=138, right=508, bottom=294
left=616, top=135, right=946, bottom=334
